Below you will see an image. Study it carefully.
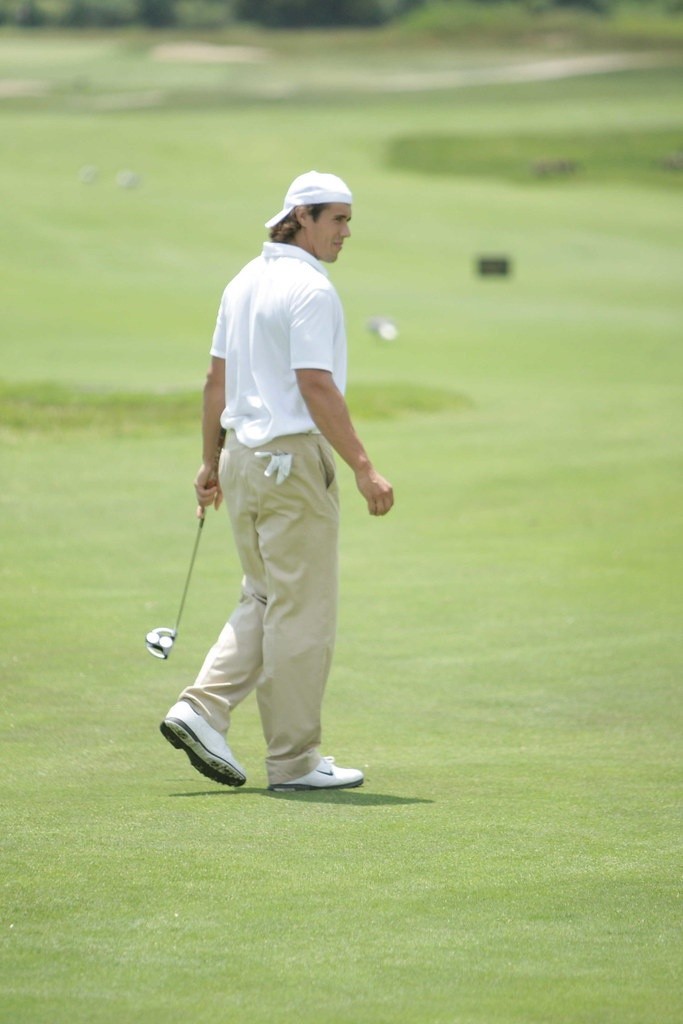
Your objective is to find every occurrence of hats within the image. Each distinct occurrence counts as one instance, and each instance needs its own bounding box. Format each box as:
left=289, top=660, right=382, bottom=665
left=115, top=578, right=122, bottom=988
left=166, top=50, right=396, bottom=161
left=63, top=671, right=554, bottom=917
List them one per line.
left=266, top=171, right=352, bottom=230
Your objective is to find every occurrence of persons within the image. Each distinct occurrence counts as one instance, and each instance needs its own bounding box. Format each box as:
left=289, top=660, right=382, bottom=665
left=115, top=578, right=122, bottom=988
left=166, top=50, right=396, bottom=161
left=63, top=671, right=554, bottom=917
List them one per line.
left=159, top=171, right=394, bottom=792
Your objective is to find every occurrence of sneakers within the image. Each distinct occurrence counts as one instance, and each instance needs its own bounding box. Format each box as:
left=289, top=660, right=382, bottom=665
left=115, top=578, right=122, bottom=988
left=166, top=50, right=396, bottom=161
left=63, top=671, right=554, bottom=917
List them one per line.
left=161, top=700, right=248, bottom=789
left=269, top=761, right=365, bottom=792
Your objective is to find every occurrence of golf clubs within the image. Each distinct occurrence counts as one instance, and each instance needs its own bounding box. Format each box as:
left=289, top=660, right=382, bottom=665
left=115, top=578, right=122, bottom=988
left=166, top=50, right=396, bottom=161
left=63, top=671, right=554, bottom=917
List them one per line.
left=143, top=425, right=228, bottom=661
